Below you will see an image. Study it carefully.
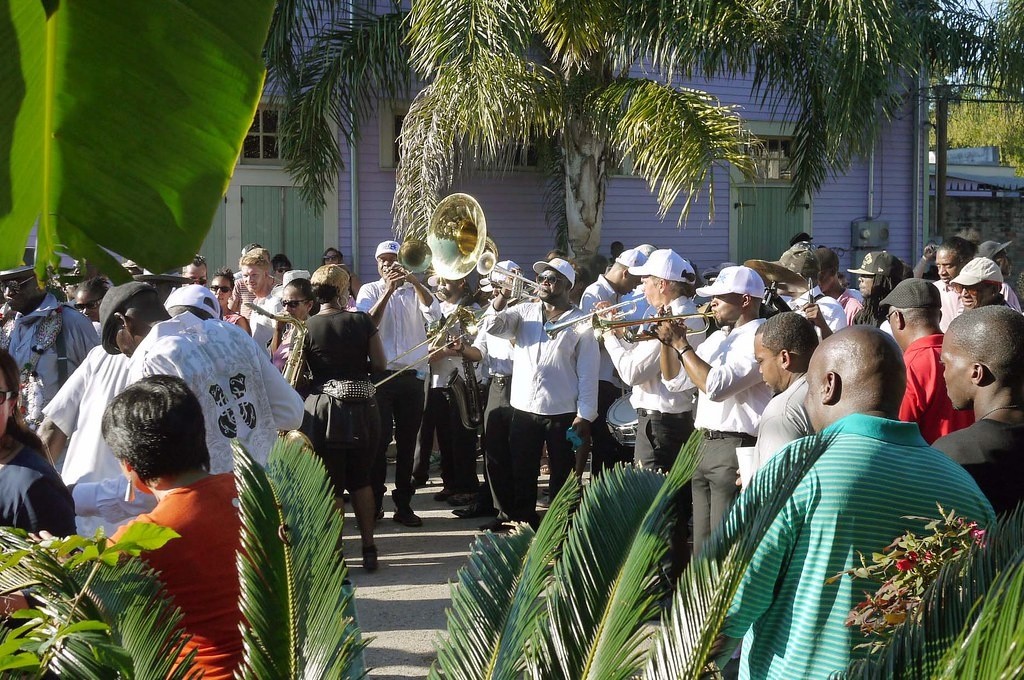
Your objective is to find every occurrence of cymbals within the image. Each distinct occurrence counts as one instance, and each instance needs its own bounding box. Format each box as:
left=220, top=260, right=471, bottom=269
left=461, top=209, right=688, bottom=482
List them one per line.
left=743, top=260, right=809, bottom=298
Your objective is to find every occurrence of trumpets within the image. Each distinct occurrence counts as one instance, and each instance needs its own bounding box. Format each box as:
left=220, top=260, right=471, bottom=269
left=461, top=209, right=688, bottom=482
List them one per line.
left=591, top=310, right=717, bottom=343
left=476, top=252, right=546, bottom=301
left=542, top=296, right=647, bottom=341
left=397, top=239, right=432, bottom=279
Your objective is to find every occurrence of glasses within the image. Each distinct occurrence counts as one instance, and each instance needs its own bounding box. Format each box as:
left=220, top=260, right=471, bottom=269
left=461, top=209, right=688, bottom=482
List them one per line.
left=0, top=276, right=34, bottom=293
left=280, top=298, right=309, bottom=308
left=74, top=296, right=103, bottom=309
left=189, top=277, right=231, bottom=294
left=108, top=322, right=120, bottom=351
left=323, top=255, right=338, bottom=260
left=536, top=273, right=569, bottom=284
left=954, top=283, right=992, bottom=295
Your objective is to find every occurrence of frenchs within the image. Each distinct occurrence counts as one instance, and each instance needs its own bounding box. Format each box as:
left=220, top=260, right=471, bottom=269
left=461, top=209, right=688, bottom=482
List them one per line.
left=427, top=193, right=499, bottom=293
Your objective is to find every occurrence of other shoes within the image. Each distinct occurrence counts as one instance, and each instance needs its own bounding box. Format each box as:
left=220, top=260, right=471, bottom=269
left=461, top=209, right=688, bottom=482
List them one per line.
left=394, top=511, right=422, bottom=527
left=361, top=544, right=379, bottom=571
left=412, top=478, right=515, bottom=532
left=535, top=488, right=550, bottom=507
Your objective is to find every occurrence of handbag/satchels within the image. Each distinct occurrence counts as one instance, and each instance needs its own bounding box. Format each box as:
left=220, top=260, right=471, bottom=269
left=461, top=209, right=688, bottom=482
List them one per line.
left=311, top=378, right=377, bottom=402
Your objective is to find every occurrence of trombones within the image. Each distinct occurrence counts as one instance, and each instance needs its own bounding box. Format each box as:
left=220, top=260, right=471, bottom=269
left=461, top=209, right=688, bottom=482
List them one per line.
left=368, top=306, right=488, bottom=388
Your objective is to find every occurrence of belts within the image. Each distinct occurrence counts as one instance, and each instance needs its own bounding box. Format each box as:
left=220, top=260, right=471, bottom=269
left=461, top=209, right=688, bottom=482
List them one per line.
left=385, top=369, right=417, bottom=376
left=636, top=407, right=693, bottom=419
left=703, top=431, right=750, bottom=439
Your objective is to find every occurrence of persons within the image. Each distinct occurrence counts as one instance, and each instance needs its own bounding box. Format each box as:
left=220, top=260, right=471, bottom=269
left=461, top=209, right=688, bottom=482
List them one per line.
left=0, top=231, right=1023, bottom=680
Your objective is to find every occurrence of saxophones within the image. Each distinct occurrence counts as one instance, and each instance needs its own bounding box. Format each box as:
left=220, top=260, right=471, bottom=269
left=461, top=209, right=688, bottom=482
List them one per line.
left=243, top=302, right=315, bottom=459
left=446, top=309, right=483, bottom=430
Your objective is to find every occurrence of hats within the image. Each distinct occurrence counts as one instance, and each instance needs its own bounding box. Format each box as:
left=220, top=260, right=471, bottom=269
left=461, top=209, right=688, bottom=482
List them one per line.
left=615, top=244, right=765, bottom=299
left=532, top=258, right=575, bottom=292
left=375, top=240, right=401, bottom=260
left=768, top=231, right=943, bottom=307
left=164, top=283, right=221, bottom=320
left=949, top=238, right=1013, bottom=286
left=0, top=249, right=37, bottom=280
left=99, top=281, right=159, bottom=355
left=479, top=259, right=521, bottom=293
